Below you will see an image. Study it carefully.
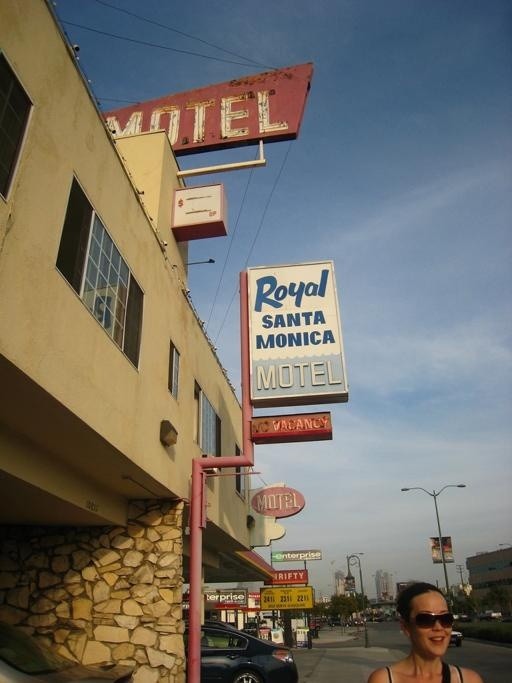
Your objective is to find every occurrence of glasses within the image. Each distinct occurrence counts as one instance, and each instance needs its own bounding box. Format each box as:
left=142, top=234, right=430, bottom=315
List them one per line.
left=407, top=612, right=455, bottom=628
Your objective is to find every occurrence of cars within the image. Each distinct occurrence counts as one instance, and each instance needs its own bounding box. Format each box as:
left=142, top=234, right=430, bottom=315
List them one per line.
left=183, top=625, right=299, bottom=683
left=328, top=617, right=351, bottom=627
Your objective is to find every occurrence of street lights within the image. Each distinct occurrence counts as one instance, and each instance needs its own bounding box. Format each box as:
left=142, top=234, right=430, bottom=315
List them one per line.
left=344, top=554, right=371, bottom=648
left=401, top=483, right=467, bottom=615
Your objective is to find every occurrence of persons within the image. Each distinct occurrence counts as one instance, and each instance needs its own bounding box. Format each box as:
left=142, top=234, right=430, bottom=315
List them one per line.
left=365, top=579, right=488, bottom=682
